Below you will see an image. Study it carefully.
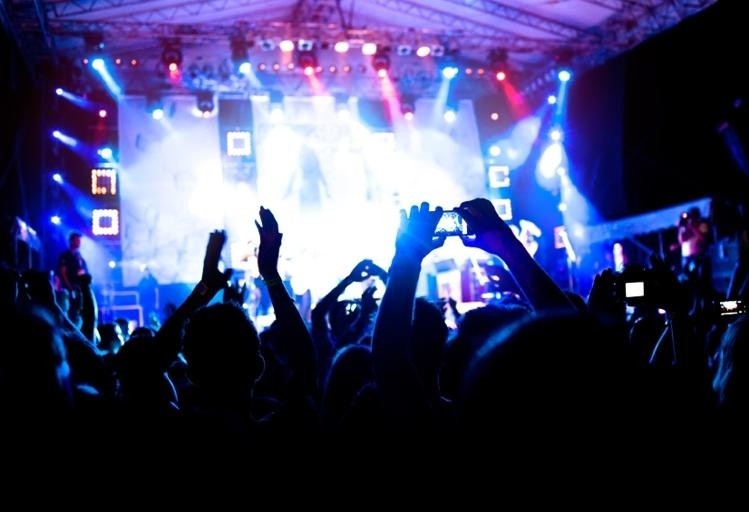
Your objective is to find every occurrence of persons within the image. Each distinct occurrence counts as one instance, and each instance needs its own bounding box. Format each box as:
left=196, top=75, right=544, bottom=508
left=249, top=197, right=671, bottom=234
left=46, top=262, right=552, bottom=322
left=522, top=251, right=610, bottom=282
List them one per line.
left=56, top=229, right=91, bottom=289
left=1, top=271, right=160, bottom=505
left=678, top=206, right=714, bottom=273
left=589, top=263, right=748, bottom=509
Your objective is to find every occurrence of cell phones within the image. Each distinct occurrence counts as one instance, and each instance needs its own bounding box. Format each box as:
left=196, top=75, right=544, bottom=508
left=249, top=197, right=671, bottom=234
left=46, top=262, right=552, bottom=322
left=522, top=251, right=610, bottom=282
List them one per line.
left=713, top=300, right=744, bottom=315
left=615, top=279, right=648, bottom=298
left=427, top=210, right=475, bottom=236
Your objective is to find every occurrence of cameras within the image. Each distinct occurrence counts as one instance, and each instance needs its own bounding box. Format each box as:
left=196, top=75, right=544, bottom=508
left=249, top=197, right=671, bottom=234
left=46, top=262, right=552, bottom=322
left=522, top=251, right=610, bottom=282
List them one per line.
left=679, top=212, right=688, bottom=235
